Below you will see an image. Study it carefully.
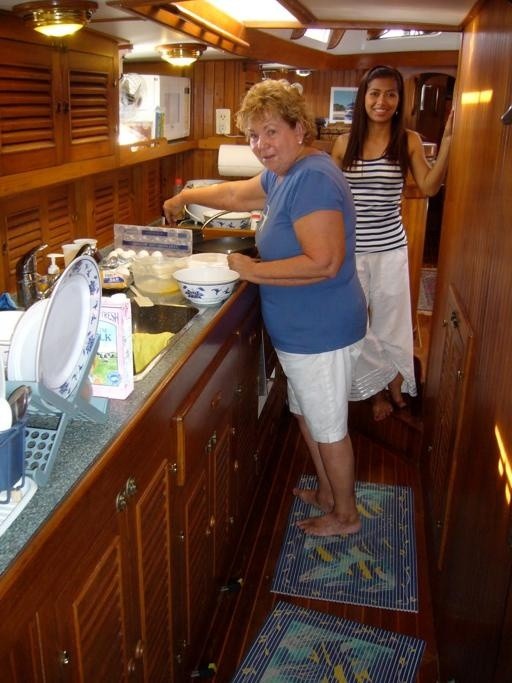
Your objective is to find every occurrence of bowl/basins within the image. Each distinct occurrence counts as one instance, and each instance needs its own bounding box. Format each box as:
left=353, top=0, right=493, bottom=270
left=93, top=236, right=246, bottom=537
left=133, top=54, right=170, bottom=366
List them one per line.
left=173, top=267, right=241, bottom=305
left=203, top=210, right=252, bottom=230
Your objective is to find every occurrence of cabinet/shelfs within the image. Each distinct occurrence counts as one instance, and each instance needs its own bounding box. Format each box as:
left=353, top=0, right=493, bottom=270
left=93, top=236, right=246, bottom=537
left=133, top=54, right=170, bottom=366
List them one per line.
left=233, top=298, right=286, bottom=518
left=171, top=334, right=238, bottom=648
left=36, top=440, right=182, bottom=683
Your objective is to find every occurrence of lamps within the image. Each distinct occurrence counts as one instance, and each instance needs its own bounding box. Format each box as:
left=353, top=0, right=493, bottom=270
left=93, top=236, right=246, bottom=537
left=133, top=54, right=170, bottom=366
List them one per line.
left=155, top=44, right=207, bottom=66
left=13, top=0, right=98, bottom=38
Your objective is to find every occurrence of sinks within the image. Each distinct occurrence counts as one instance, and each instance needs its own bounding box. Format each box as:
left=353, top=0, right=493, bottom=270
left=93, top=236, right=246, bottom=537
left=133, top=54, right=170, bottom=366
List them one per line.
left=131, top=300, right=210, bottom=380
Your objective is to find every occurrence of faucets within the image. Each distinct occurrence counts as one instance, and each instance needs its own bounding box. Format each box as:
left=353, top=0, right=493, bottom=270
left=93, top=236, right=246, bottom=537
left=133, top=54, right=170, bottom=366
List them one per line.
left=16, top=243, right=100, bottom=314
left=200, top=209, right=235, bottom=230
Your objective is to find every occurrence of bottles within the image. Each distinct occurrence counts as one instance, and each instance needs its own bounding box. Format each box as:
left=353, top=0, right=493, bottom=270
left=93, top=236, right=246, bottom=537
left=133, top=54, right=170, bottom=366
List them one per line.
left=173, top=177, right=185, bottom=220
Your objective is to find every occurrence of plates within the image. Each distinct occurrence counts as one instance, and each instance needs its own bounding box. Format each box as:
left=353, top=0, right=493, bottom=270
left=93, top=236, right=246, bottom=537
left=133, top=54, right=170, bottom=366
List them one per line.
left=6, top=297, right=50, bottom=382
left=36, top=255, right=102, bottom=407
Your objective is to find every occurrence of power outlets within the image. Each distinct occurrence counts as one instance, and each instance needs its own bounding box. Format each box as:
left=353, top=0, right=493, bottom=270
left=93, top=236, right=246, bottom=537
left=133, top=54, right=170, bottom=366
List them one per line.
left=215, top=108, right=231, bottom=134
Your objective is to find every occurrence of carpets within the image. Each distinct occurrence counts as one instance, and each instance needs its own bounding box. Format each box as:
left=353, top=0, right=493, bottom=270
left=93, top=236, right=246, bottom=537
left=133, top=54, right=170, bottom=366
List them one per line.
left=417, top=267, right=437, bottom=316
left=231, top=600, right=427, bottom=683
left=269, top=473, right=420, bottom=614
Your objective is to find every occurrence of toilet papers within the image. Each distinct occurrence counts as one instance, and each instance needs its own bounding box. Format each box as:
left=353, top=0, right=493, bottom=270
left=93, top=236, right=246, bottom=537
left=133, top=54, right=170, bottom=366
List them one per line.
left=217, top=144, right=266, bottom=177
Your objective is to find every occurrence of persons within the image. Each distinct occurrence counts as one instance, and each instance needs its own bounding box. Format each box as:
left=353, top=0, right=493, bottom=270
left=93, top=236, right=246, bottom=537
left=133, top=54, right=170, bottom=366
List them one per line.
left=163, top=78, right=371, bottom=537
left=327, top=63, right=455, bottom=420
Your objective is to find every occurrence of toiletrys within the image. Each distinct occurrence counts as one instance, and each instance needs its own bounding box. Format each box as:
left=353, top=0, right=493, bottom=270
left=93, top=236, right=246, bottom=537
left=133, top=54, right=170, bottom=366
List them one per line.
left=46, top=253, right=64, bottom=282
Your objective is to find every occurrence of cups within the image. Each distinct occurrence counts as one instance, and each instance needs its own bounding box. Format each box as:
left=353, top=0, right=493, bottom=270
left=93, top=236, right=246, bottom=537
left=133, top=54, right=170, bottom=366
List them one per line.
left=62, top=238, right=99, bottom=268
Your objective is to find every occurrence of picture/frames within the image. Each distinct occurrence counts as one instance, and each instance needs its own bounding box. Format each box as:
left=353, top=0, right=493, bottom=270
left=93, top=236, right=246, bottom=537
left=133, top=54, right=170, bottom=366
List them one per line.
left=329, top=86, right=359, bottom=124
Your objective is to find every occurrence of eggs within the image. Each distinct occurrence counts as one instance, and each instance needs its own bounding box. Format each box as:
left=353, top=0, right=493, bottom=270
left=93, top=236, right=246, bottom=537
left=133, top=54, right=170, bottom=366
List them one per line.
left=107, top=248, right=163, bottom=259
